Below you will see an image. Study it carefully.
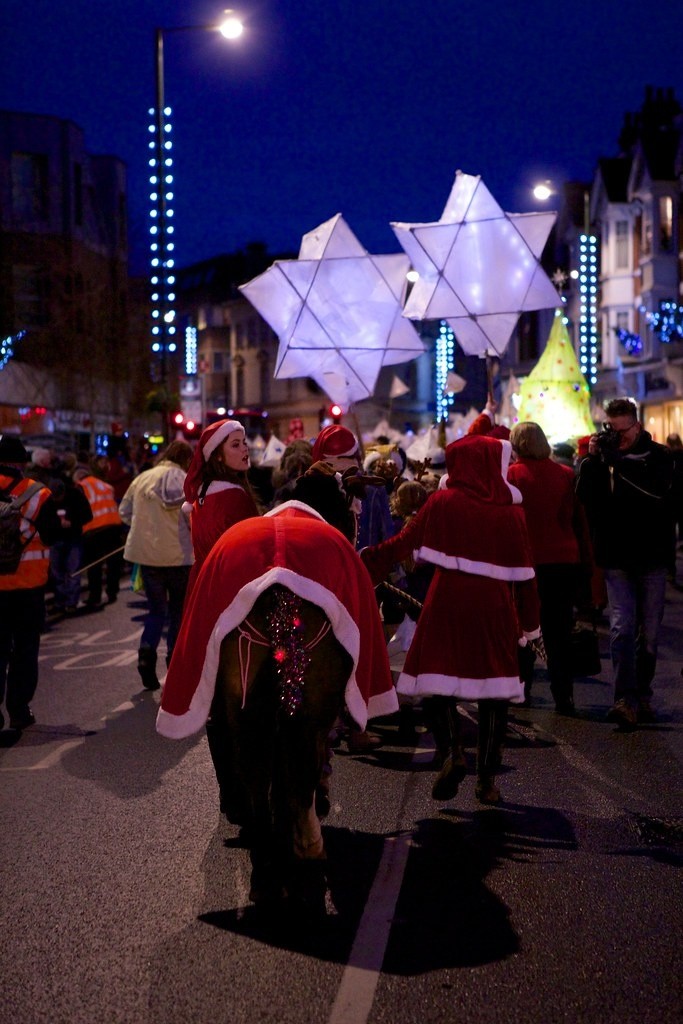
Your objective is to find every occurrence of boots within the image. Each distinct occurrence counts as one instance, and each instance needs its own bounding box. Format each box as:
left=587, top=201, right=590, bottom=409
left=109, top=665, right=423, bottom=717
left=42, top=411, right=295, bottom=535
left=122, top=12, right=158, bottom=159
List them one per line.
left=427, top=703, right=469, bottom=801
left=472, top=732, right=503, bottom=805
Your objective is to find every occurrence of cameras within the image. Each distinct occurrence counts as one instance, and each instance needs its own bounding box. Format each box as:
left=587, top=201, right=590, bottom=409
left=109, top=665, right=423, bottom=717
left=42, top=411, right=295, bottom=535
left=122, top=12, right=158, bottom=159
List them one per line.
left=598, top=430, right=621, bottom=452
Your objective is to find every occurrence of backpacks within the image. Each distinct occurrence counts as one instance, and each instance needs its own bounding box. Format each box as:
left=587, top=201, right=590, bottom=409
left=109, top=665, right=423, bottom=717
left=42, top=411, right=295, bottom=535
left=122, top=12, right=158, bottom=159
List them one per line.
left=0, top=482, right=45, bottom=574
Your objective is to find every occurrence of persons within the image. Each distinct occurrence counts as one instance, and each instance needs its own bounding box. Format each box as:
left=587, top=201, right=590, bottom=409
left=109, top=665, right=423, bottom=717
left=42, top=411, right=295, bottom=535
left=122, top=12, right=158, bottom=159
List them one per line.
left=0, top=433, right=71, bottom=730
left=27, top=398, right=683, bottom=800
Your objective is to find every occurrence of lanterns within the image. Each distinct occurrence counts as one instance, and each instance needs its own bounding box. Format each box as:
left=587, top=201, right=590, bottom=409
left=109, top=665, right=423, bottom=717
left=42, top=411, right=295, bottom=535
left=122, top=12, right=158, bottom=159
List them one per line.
left=390, top=170, right=564, bottom=356
left=240, top=214, right=428, bottom=413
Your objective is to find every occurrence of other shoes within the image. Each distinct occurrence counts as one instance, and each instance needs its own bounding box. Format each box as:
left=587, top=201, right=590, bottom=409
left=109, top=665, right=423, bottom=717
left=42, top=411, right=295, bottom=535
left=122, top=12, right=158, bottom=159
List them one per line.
left=508, top=689, right=529, bottom=707
left=351, top=730, right=371, bottom=748
left=639, top=697, right=657, bottom=719
left=137, top=647, right=161, bottom=690
left=9, top=708, right=36, bottom=730
left=607, top=699, right=637, bottom=727
left=65, top=606, right=75, bottom=613
left=555, top=697, right=574, bottom=713
left=108, top=593, right=117, bottom=602
left=82, top=597, right=101, bottom=604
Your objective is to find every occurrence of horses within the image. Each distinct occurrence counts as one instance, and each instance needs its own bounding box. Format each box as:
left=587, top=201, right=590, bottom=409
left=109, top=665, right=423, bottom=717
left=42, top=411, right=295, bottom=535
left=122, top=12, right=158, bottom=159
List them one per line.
left=205, top=461, right=384, bottom=886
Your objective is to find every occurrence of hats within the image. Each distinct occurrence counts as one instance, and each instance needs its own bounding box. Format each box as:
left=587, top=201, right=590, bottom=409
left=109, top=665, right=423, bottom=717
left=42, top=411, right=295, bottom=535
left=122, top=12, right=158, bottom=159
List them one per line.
left=425, top=448, right=446, bottom=470
left=375, top=444, right=402, bottom=475
left=437, top=473, right=448, bottom=489
left=0, top=433, right=28, bottom=464
left=363, top=450, right=382, bottom=475
left=181, top=418, right=245, bottom=513
left=311, top=425, right=358, bottom=464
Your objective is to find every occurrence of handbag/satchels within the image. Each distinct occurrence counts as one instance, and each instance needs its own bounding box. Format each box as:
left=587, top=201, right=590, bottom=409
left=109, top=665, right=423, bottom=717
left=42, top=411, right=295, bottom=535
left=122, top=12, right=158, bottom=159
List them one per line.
left=127, top=563, right=144, bottom=593
left=592, top=569, right=608, bottom=608
left=576, top=637, right=602, bottom=676
